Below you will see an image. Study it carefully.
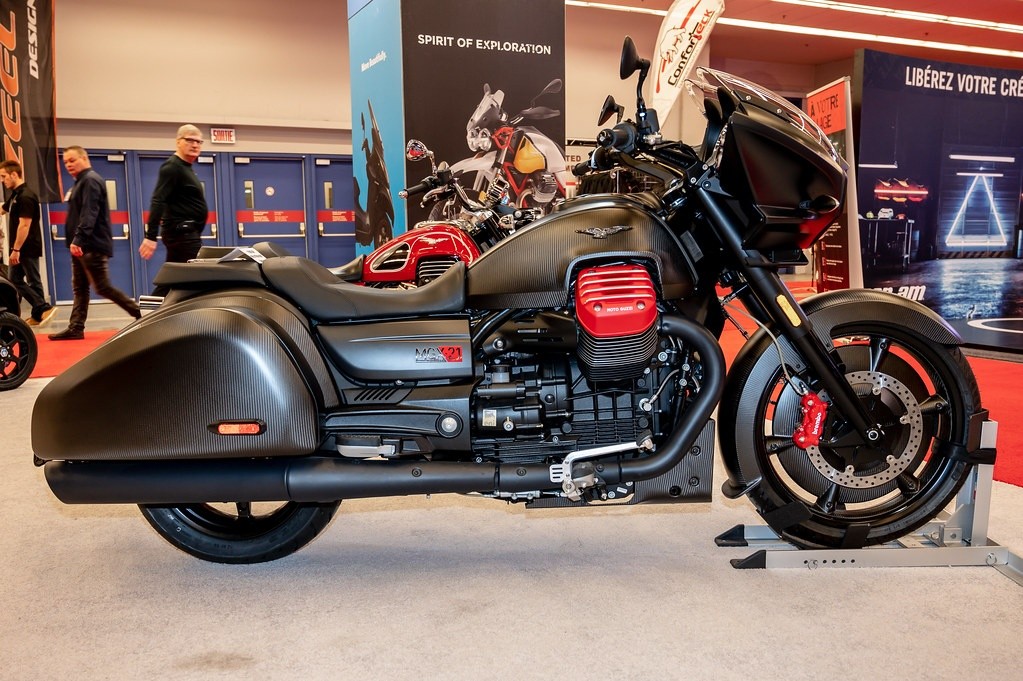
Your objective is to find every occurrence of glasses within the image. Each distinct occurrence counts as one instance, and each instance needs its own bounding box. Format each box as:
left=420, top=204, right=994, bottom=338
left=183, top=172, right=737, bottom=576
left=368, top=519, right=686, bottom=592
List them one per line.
left=180, top=137, right=205, bottom=146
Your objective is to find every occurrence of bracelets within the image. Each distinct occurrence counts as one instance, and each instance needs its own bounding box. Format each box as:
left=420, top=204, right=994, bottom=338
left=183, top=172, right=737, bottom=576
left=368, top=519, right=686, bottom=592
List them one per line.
left=11, top=247, right=20, bottom=252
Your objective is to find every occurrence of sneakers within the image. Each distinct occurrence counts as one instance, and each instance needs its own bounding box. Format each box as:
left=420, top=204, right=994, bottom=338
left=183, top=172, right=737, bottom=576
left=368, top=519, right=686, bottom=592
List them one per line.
left=24, top=317, right=40, bottom=327
left=38, top=306, right=57, bottom=327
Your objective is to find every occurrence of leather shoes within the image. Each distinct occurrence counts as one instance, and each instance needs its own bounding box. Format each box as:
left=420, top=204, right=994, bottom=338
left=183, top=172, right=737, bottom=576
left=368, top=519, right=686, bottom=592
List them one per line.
left=49, top=326, right=86, bottom=341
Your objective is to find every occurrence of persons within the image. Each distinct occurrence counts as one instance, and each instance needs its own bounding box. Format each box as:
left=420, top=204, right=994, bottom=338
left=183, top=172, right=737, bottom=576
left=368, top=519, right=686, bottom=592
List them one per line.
left=139, top=122, right=210, bottom=305
left=47, top=145, right=143, bottom=342
left=0, top=160, right=58, bottom=329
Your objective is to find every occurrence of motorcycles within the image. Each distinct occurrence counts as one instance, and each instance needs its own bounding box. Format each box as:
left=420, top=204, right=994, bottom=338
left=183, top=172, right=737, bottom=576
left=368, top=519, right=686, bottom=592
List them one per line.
left=0, top=272, right=38, bottom=390
left=31, top=36, right=988, bottom=563
